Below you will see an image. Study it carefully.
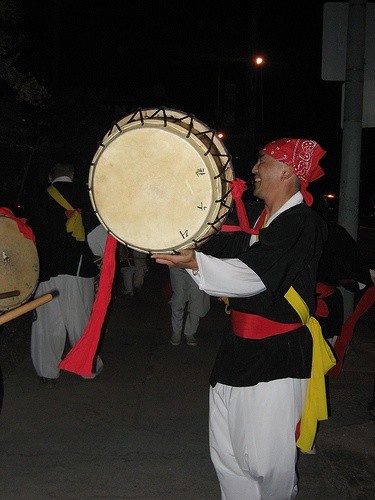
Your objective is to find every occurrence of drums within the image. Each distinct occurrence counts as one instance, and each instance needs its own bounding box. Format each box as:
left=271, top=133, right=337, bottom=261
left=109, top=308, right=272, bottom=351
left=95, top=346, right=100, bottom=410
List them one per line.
left=0, top=216, right=39, bottom=312
left=86, top=107, right=237, bottom=256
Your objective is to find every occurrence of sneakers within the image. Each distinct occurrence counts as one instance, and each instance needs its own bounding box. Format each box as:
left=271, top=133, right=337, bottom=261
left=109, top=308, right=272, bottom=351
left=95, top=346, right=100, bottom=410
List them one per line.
left=170, top=335, right=181, bottom=346
left=123, top=289, right=134, bottom=296
left=185, top=335, right=198, bottom=346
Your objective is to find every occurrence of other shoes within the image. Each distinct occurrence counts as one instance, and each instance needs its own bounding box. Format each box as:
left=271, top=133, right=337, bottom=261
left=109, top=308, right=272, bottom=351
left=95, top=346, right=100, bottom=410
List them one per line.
left=305, top=444, right=316, bottom=454
left=80, top=377, right=94, bottom=384
left=40, top=377, right=56, bottom=389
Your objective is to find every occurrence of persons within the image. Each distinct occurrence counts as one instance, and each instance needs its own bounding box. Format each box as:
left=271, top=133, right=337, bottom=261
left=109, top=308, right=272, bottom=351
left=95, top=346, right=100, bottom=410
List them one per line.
left=119, top=244, right=148, bottom=295
left=300, top=223, right=372, bottom=455
left=168, top=246, right=217, bottom=346
left=152, top=137, right=326, bottom=500
left=20, top=162, right=108, bottom=388
